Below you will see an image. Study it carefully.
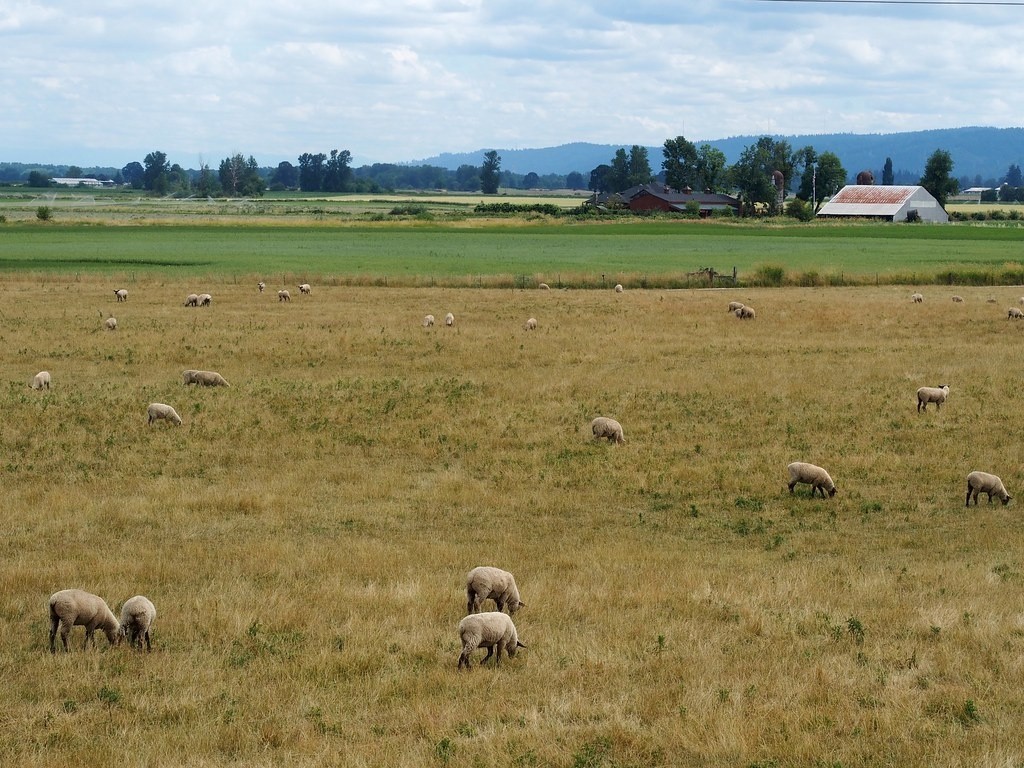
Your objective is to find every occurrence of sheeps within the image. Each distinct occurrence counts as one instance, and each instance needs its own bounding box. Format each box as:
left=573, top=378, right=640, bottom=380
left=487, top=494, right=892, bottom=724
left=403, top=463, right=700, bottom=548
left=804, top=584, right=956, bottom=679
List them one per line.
left=525, top=318, right=537, bottom=331
left=786, top=463, right=838, bottom=499
left=106, top=318, right=116, bottom=330
left=615, top=285, right=622, bottom=293
left=28, top=371, right=50, bottom=391
left=119, top=595, right=155, bottom=653
left=147, top=402, right=182, bottom=428
left=258, top=282, right=311, bottom=301
left=965, top=471, right=1013, bottom=506
left=916, top=385, right=950, bottom=413
left=538, top=284, right=550, bottom=291
left=912, top=293, right=1024, bottom=321
left=113, top=289, right=128, bottom=302
left=591, top=417, right=626, bottom=445
left=179, top=370, right=231, bottom=388
left=48, top=589, right=126, bottom=653
left=422, top=313, right=454, bottom=328
left=457, top=566, right=528, bottom=669
left=183, top=294, right=212, bottom=307
left=727, top=302, right=755, bottom=321
